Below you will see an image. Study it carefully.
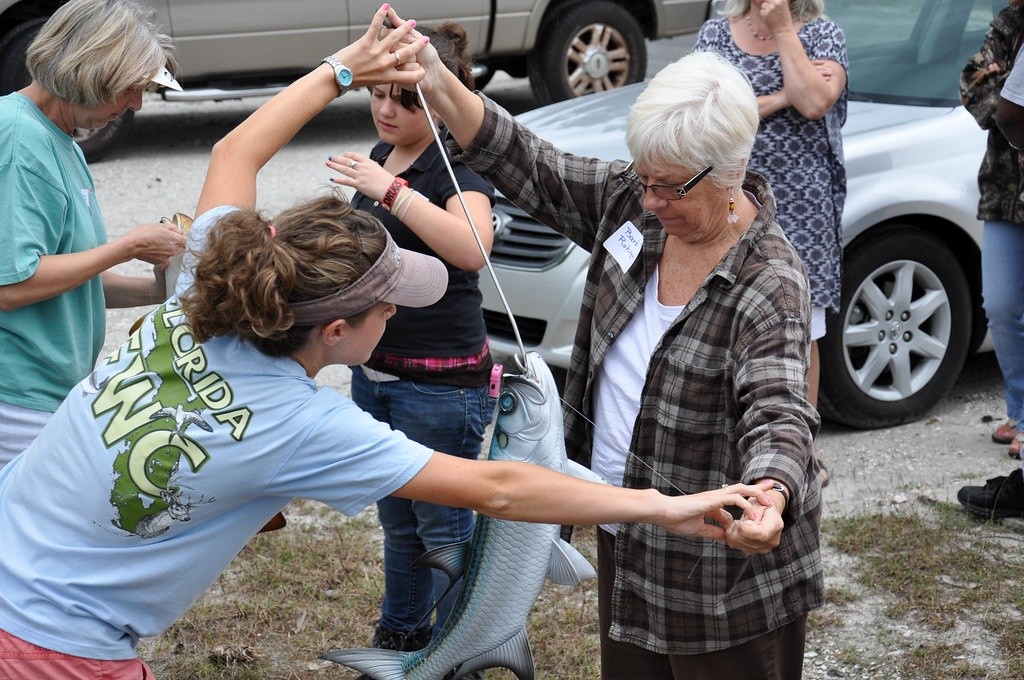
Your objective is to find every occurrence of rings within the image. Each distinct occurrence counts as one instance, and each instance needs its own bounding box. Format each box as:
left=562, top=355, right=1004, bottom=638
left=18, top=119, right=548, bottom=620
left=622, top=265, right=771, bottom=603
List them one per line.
left=393, top=54, right=403, bottom=65
left=722, top=482, right=728, bottom=491
left=351, top=160, right=357, bottom=168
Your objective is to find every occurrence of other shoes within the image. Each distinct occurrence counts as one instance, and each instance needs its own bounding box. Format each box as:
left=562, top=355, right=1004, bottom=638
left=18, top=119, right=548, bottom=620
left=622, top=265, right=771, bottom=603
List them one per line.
left=356, top=627, right=486, bottom=680
left=992, top=419, right=1024, bottom=459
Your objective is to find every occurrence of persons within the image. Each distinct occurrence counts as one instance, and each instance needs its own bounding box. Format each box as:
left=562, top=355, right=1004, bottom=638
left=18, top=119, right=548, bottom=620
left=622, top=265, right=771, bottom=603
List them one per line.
left=349, top=22, right=502, bottom=651
left=957, top=41, right=1024, bottom=515
left=960, top=0, right=1023, bottom=456
left=0, top=4, right=779, bottom=679
left=379, top=8, right=822, bottom=680
left=1, top=0, right=188, bottom=474
left=693, top=0, right=849, bottom=487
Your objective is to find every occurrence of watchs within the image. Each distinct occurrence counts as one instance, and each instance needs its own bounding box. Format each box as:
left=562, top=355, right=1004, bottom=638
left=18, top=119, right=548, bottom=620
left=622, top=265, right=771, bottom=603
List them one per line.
left=318, top=54, right=352, bottom=100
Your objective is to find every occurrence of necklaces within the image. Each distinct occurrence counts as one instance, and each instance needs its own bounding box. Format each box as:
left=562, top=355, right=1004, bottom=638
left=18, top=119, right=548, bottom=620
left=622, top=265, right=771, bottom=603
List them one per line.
left=748, top=15, right=774, bottom=41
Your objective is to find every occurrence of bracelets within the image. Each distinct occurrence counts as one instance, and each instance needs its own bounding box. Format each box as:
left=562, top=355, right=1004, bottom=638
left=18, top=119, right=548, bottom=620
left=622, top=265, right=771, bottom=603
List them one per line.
left=771, top=481, right=790, bottom=511
left=381, top=176, right=417, bottom=220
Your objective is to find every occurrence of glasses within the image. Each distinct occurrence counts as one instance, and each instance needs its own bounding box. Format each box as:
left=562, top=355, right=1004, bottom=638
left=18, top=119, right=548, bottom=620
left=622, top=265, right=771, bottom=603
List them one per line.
left=620, top=159, right=713, bottom=201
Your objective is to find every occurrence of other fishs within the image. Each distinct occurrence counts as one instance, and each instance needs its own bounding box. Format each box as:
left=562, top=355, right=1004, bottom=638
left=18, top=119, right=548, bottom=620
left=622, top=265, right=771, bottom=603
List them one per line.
left=319, top=351, right=626, bottom=679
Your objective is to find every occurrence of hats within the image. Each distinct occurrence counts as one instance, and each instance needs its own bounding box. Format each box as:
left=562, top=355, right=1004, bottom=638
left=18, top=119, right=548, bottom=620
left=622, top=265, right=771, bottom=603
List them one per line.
left=291, top=217, right=448, bottom=322
left=142, top=64, right=182, bottom=92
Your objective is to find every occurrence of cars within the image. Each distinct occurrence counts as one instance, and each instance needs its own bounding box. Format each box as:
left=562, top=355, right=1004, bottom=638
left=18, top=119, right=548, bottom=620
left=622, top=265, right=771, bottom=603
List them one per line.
left=0, top=1, right=716, bottom=163
left=477, top=2, right=1024, bottom=431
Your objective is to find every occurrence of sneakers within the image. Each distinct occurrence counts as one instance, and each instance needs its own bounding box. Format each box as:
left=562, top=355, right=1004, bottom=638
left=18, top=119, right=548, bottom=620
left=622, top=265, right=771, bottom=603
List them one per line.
left=958, top=468, right=1024, bottom=518
